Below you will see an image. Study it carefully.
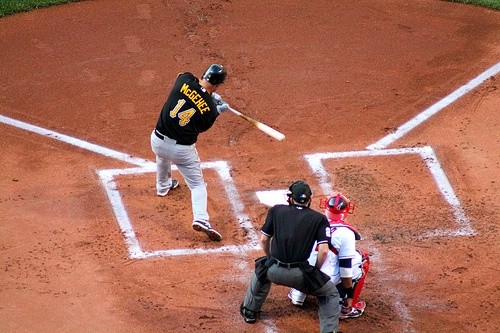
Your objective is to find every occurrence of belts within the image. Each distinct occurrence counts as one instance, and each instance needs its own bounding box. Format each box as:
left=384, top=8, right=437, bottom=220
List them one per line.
left=273, top=260, right=299, bottom=268
left=155, top=129, right=181, bottom=144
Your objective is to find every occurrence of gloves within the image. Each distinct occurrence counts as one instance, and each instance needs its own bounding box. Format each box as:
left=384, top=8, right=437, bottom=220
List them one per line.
left=211, top=92, right=222, bottom=105
left=216, top=103, right=229, bottom=114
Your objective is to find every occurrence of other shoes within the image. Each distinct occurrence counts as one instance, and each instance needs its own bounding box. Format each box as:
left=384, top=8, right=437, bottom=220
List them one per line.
left=170, top=179, right=179, bottom=189
left=192, top=220, right=222, bottom=242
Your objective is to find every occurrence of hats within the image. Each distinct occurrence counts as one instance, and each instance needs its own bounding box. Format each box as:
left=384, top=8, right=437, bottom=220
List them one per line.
left=291, top=182, right=309, bottom=204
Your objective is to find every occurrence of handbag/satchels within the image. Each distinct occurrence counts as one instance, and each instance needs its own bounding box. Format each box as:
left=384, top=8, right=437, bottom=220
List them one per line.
left=298, top=263, right=330, bottom=294
left=255, top=256, right=274, bottom=285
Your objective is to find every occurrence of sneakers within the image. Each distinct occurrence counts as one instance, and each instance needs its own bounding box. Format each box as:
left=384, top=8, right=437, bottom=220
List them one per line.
left=339, top=305, right=364, bottom=320
left=240, top=302, right=256, bottom=322
left=287, top=288, right=306, bottom=305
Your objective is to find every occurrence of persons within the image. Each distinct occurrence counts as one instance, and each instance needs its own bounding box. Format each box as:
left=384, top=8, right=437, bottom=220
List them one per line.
left=151, top=63, right=228, bottom=242
left=287, top=195, right=370, bottom=320
left=239, top=181, right=343, bottom=333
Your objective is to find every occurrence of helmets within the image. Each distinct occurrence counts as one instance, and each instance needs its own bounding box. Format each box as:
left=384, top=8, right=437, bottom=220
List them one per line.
left=202, top=63, right=226, bottom=85
left=326, top=194, right=350, bottom=215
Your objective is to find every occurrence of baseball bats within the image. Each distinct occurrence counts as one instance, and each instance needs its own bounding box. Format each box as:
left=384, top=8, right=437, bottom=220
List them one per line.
left=217, top=100, right=286, bottom=142
